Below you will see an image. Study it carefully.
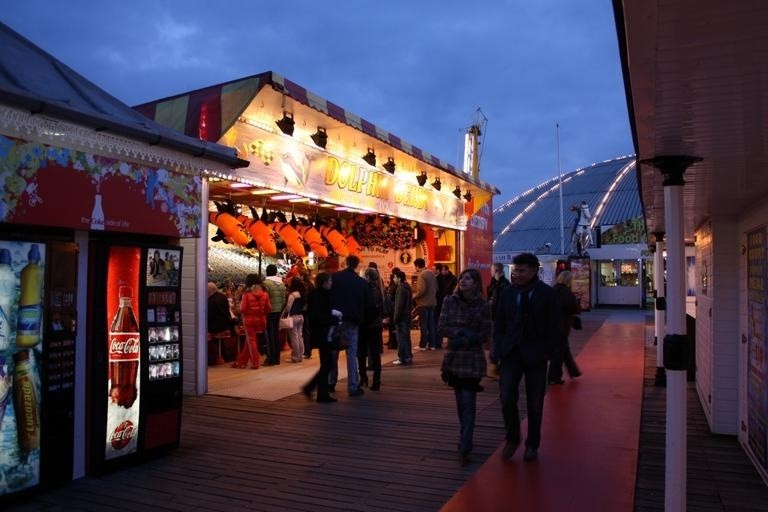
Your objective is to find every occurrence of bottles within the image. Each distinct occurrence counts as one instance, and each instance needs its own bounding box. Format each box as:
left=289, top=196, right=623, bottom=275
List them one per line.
left=108, top=284, right=138, bottom=408
left=9, top=351, right=39, bottom=454
left=17, top=244, right=42, bottom=347
left=0, top=248, right=16, bottom=348
left=0, top=356, right=12, bottom=431
left=89, top=193, right=106, bottom=231
left=148, top=304, right=180, bottom=381
left=49, top=290, right=74, bottom=389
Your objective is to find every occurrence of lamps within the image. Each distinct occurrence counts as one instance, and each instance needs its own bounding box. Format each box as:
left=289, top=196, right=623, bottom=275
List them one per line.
left=274, top=112, right=472, bottom=203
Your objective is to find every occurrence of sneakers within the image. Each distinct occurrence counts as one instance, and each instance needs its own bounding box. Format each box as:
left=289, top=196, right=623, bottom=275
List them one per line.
left=502, top=439, right=521, bottom=461
left=303, top=382, right=380, bottom=403
left=523, top=446, right=538, bottom=461
left=413, top=346, right=437, bottom=350
left=230, top=354, right=313, bottom=369
left=392, top=359, right=404, bottom=365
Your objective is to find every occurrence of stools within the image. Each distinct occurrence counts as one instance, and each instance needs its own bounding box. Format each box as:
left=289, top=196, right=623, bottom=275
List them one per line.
left=237, top=327, right=257, bottom=356
left=213, top=331, right=231, bottom=364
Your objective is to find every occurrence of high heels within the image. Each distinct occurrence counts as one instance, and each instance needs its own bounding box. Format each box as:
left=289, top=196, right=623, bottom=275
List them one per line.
left=547, top=373, right=565, bottom=385
left=570, top=372, right=582, bottom=379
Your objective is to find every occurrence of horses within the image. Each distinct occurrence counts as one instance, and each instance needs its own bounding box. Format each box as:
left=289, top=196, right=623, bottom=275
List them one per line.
left=568, top=200, right=595, bottom=254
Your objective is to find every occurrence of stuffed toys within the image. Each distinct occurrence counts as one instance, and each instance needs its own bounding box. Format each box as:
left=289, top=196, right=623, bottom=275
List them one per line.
left=208, top=198, right=363, bottom=259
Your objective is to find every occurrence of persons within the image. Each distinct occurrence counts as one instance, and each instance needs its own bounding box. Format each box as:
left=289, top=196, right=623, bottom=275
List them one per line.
left=489, top=253, right=562, bottom=465
left=548, top=270, right=582, bottom=384
left=207, top=252, right=315, bottom=370
left=434, top=268, right=492, bottom=464
left=302, top=255, right=384, bottom=402
left=384, top=258, right=436, bottom=366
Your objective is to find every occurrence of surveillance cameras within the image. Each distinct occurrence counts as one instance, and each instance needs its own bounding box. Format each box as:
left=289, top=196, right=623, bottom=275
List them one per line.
left=545, top=243, right=555, bottom=250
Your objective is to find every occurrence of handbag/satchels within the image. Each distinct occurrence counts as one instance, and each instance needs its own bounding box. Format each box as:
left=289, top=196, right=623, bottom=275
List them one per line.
left=278, top=308, right=294, bottom=331
